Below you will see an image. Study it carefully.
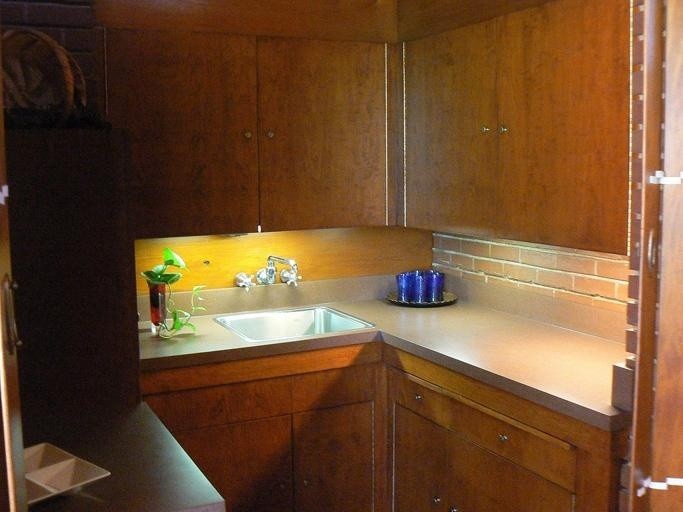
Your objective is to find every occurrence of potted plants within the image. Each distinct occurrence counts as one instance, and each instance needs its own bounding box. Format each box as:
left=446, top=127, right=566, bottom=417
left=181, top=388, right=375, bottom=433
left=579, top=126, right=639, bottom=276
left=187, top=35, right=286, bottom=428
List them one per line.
left=140, top=247, right=208, bottom=338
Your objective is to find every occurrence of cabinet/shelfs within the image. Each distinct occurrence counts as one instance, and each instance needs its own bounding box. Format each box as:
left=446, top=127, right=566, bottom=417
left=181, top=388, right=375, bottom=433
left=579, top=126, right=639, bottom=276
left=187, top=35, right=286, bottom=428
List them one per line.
left=0, top=342, right=612, bottom=512
left=79, top=0, right=645, bottom=260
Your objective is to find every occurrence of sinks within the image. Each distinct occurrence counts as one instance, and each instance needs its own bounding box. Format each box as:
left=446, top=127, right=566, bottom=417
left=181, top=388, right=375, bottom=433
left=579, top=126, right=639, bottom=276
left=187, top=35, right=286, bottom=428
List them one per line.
left=213, top=305, right=375, bottom=344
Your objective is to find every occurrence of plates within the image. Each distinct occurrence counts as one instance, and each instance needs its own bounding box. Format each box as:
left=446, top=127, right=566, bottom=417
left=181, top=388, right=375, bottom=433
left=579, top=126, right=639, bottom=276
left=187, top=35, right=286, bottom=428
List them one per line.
left=387, top=291, right=457, bottom=306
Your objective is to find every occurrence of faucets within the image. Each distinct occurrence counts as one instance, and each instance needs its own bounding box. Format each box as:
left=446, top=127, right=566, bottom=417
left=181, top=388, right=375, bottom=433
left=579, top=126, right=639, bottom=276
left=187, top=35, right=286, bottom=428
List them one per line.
left=265, top=256, right=298, bottom=279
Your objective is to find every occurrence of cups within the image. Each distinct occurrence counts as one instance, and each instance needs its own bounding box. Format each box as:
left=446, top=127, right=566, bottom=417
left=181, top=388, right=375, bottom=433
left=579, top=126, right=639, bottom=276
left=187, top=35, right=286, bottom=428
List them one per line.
left=396, top=270, right=443, bottom=302
